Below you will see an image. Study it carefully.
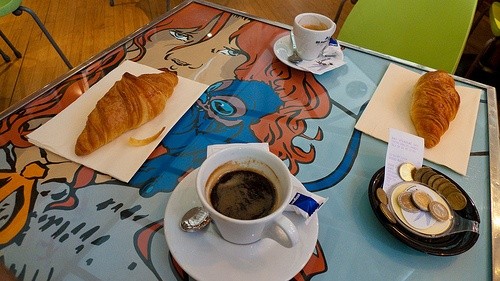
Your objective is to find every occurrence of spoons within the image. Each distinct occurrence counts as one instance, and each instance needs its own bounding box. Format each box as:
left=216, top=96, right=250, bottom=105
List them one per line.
left=288, top=30, right=303, bottom=65
left=180, top=207, right=211, bottom=234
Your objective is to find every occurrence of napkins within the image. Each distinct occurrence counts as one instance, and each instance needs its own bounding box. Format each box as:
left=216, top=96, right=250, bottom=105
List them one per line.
left=355, top=62, right=484, bottom=176
left=23, top=60, right=209, bottom=185
left=204, top=142, right=270, bottom=158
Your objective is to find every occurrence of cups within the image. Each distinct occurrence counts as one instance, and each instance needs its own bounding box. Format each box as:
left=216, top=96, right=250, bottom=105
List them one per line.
left=294, top=12, right=337, bottom=61
left=196, top=146, right=299, bottom=248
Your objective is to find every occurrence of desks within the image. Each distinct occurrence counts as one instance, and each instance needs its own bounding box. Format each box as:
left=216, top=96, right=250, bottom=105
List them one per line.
left=0, top=0, right=500, bottom=281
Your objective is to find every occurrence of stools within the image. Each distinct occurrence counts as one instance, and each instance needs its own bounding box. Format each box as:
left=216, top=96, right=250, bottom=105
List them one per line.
left=0, top=0, right=73, bottom=70
left=464, top=0, right=500, bottom=79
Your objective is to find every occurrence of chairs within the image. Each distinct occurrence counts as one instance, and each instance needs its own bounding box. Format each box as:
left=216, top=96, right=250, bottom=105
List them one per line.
left=336, top=0, right=478, bottom=75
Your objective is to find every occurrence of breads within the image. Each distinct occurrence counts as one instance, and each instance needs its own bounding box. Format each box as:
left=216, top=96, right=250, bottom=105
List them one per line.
left=410, top=70, right=461, bottom=148
left=75, top=71, right=179, bottom=155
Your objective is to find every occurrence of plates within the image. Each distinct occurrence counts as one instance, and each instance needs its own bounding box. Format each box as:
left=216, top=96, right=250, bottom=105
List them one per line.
left=162, top=166, right=319, bottom=281
left=273, top=34, right=344, bottom=72
left=368, top=165, right=481, bottom=257
